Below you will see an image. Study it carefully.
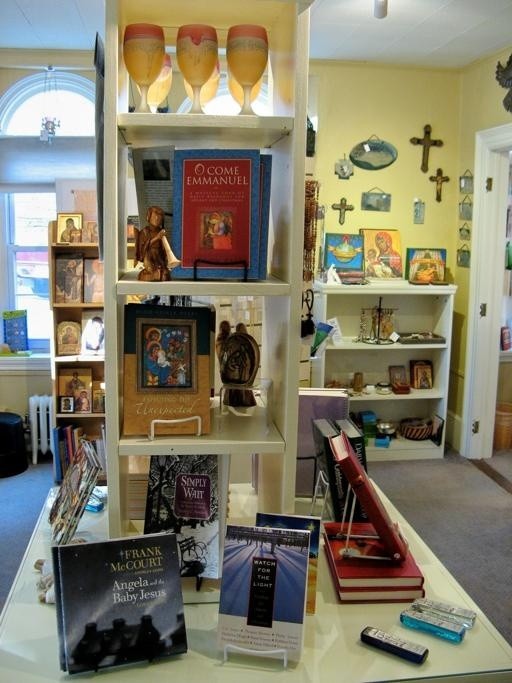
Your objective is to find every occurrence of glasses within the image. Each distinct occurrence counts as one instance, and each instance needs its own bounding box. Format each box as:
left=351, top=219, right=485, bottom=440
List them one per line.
left=374, top=0, right=388, bottom=18
left=1, top=65, right=98, bottom=148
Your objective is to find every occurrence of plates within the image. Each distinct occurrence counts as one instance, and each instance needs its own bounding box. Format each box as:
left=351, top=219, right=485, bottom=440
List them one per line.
left=311, top=279, right=458, bottom=463
left=48, top=220, right=107, bottom=486
left=103, top=0, right=311, bottom=604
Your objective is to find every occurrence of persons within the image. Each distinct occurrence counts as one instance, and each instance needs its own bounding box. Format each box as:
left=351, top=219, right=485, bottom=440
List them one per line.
left=74, top=391, right=89, bottom=411
left=67, top=372, right=86, bottom=396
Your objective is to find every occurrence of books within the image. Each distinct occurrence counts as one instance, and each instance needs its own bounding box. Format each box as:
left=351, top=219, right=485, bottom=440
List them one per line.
left=3, top=310, right=29, bottom=351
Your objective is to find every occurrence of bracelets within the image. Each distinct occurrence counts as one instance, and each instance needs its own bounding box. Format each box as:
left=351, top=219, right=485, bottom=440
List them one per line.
left=376, top=422, right=397, bottom=435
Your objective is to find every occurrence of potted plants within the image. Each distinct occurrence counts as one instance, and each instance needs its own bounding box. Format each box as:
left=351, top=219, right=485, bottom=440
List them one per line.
left=359, top=229, right=403, bottom=280
left=457, top=244, right=471, bottom=268
left=56, top=212, right=83, bottom=245
left=324, top=233, right=368, bottom=285
left=57, top=368, right=93, bottom=414
left=405, top=248, right=449, bottom=285
left=59, top=396, right=75, bottom=413
left=460, top=169, right=474, bottom=193
left=389, top=366, right=407, bottom=385
left=410, top=360, right=433, bottom=389
left=460, top=222, right=471, bottom=240
left=54, top=321, right=81, bottom=355
left=459, top=193, right=474, bottom=221
left=56, top=395, right=74, bottom=413
left=361, top=187, right=391, bottom=212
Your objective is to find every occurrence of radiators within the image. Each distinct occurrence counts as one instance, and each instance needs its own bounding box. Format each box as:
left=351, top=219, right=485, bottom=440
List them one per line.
left=400, top=609, right=465, bottom=644
left=92, top=487, right=107, bottom=502
left=85, top=499, right=103, bottom=511
left=412, top=596, right=477, bottom=629
left=361, top=626, right=430, bottom=665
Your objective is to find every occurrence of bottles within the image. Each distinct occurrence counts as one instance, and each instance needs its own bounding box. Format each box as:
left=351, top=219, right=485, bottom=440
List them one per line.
left=147, top=341, right=162, bottom=351
left=146, top=328, right=162, bottom=341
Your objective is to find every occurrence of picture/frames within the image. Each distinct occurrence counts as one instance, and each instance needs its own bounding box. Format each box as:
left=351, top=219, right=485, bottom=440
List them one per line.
left=0, top=478, right=511, bottom=683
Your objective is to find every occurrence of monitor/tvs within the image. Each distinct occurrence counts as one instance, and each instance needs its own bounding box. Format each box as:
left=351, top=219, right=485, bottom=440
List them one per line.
left=144, top=455, right=230, bottom=580
left=410, top=359, right=434, bottom=389
left=46, top=438, right=103, bottom=546
left=122, top=304, right=215, bottom=436
left=56, top=365, right=105, bottom=414
left=311, top=416, right=371, bottom=521
left=50, top=532, right=188, bottom=676
left=253, top=512, right=323, bottom=615
left=214, top=524, right=311, bottom=662
left=3, top=310, right=28, bottom=352
left=322, top=521, right=426, bottom=605
left=55, top=257, right=105, bottom=303
left=390, top=365, right=407, bottom=385
left=57, top=308, right=106, bottom=355
left=323, top=232, right=365, bottom=284
left=52, top=422, right=107, bottom=485
left=359, top=229, right=404, bottom=279
left=406, top=247, right=446, bottom=282
left=131, top=146, right=272, bottom=282
left=328, top=429, right=411, bottom=566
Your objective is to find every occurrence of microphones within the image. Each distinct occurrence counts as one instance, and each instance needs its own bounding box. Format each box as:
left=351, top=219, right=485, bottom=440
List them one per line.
left=0, top=412, right=29, bottom=480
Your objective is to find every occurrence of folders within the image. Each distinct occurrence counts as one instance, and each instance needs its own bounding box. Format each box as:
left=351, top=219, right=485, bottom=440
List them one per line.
left=29, top=394, right=53, bottom=465
